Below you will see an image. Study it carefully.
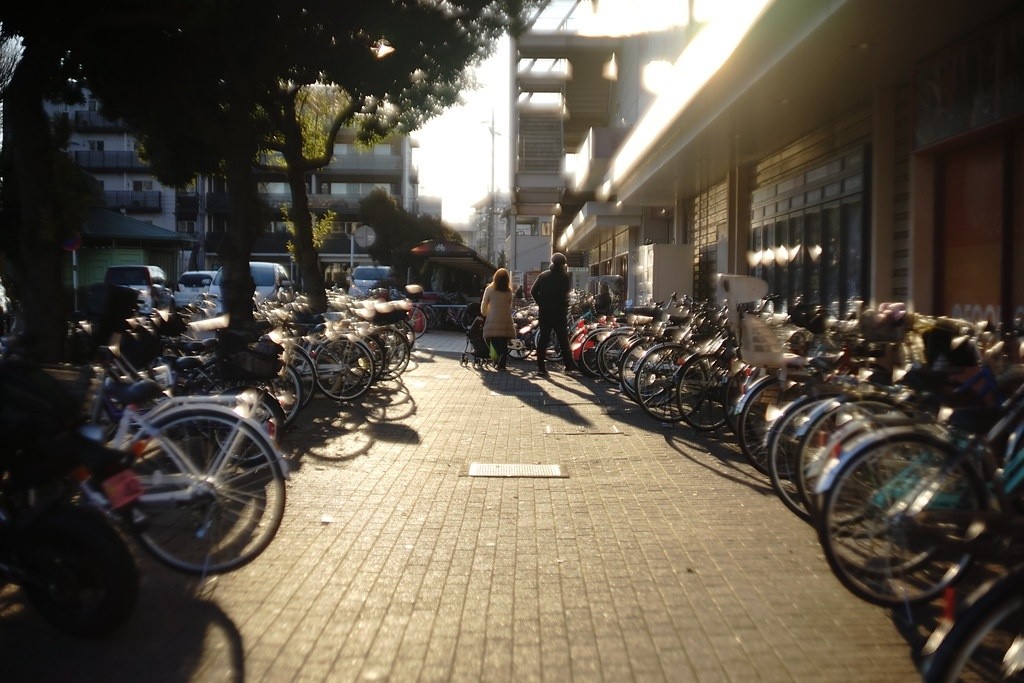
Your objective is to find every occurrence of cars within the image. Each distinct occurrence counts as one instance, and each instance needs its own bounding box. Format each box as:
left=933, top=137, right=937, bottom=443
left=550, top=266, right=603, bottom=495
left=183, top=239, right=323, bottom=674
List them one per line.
left=346, top=265, right=392, bottom=298
left=205, top=262, right=296, bottom=316
left=168, top=269, right=218, bottom=310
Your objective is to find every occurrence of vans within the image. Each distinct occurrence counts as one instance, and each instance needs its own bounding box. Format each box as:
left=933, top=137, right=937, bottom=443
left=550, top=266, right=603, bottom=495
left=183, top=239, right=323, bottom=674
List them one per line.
left=101, top=263, right=176, bottom=316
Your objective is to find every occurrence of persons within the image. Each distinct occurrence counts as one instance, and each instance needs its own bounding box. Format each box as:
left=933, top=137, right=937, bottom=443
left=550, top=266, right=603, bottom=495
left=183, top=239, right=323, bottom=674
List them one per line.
left=514, top=284, right=524, bottom=299
left=530, top=253, right=580, bottom=376
left=589, top=286, right=612, bottom=315
left=478, top=268, right=515, bottom=372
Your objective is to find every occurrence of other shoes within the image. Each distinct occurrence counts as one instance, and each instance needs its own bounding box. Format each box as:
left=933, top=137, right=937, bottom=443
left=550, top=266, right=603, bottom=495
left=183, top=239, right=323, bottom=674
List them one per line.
left=565, top=366, right=580, bottom=373
left=538, top=369, right=549, bottom=375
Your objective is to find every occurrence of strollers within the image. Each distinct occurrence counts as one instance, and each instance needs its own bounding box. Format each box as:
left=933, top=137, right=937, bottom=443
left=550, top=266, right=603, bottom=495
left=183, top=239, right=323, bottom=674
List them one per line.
left=460, top=302, right=503, bottom=371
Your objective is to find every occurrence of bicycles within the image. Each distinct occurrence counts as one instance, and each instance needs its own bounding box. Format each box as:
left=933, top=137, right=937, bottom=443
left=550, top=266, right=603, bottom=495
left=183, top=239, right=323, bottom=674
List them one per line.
left=503, top=274, right=1024, bottom=682
left=0, top=280, right=473, bottom=640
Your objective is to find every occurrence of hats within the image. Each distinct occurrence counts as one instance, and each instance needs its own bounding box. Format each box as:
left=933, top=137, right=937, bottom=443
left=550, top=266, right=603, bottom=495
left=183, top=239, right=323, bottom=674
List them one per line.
left=551, top=253, right=568, bottom=266
